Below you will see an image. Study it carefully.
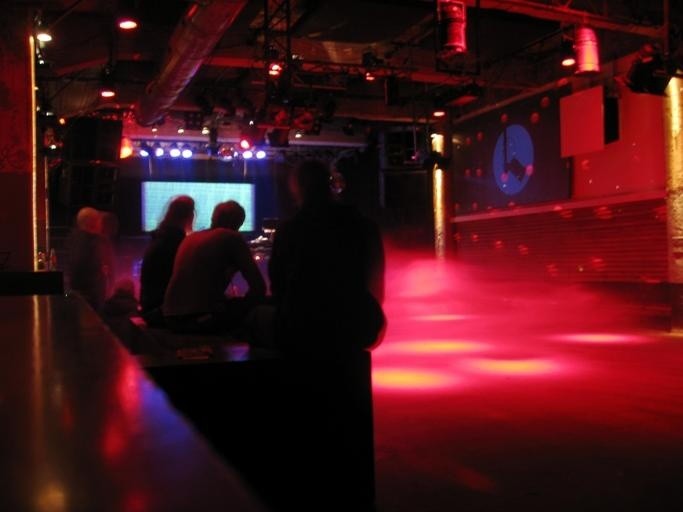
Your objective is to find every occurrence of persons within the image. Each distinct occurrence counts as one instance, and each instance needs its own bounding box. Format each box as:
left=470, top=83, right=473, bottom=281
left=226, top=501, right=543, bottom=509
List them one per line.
left=260, top=158, right=385, bottom=350
left=161, top=199, right=269, bottom=343
left=328, top=146, right=378, bottom=213
left=137, top=195, right=196, bottom=333
left=106, top=275, right=141, bottom=352
left=55, top=206, right=111, bottom=307
